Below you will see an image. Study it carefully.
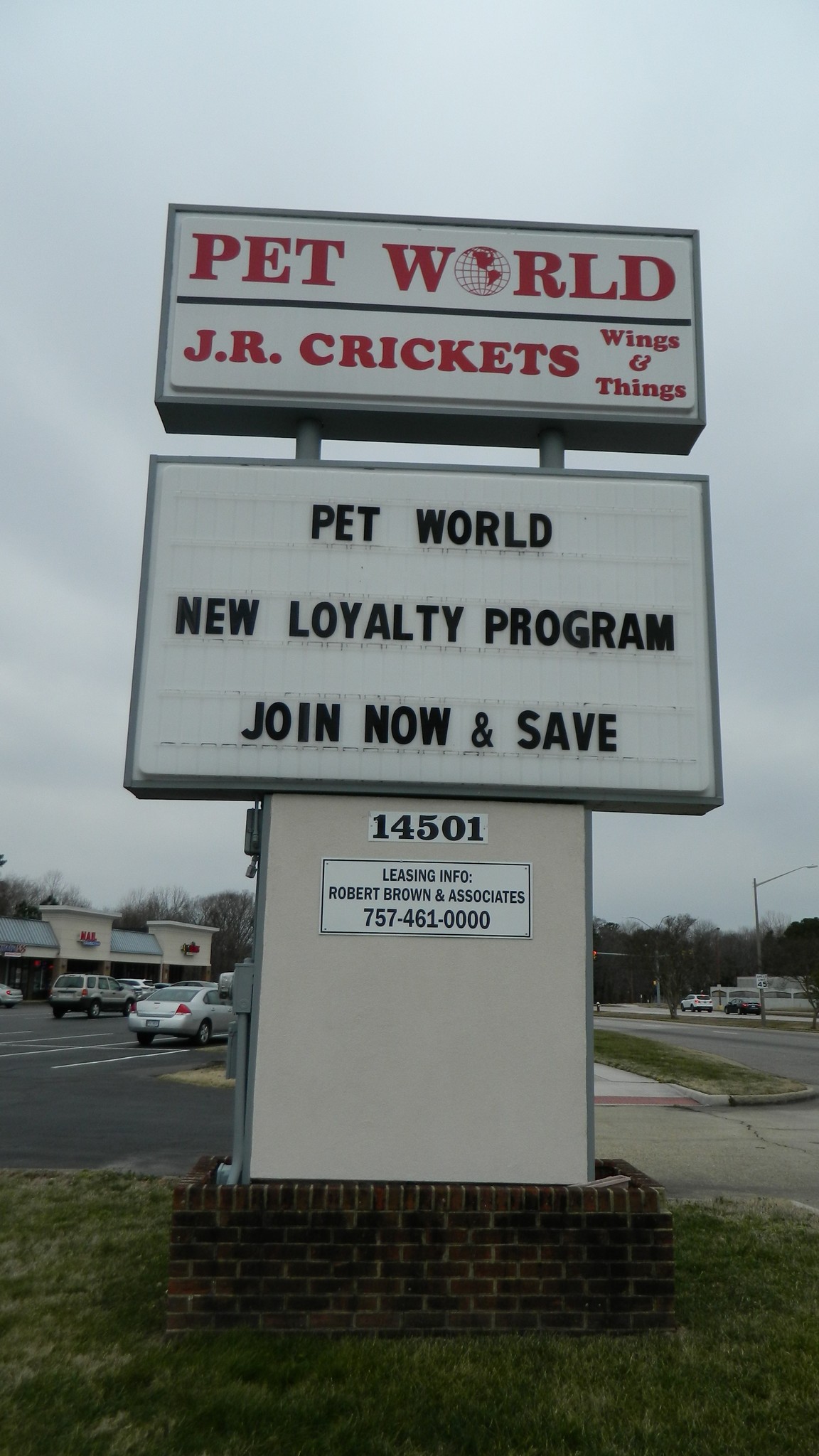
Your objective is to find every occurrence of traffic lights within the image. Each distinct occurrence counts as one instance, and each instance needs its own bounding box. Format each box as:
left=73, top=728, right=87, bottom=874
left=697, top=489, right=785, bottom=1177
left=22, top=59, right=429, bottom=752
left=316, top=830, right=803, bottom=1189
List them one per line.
left=593, top=951, right=598, bottom=960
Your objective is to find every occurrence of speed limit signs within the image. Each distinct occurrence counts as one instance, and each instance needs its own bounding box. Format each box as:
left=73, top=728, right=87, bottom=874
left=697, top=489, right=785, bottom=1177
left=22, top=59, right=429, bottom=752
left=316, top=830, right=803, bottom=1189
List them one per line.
left=756, top=974, right=769, bottom=989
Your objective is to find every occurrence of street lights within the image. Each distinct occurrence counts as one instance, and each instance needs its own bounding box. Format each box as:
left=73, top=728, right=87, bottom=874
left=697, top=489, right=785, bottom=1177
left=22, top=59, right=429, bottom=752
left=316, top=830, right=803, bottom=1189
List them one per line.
left=622, top=915, right=671, bottom=1004
left=754, top=864, right=818, bottom=1024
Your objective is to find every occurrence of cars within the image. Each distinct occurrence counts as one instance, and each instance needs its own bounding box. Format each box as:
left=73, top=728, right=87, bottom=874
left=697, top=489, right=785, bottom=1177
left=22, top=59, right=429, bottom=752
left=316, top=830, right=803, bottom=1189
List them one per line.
left=724, top=998, right=762, bottom=1015
left=0, top=984, right=23, bottom=1008
left=173, top=980, right=219, bottom=991
left=129, top=986, right=233, bottom=1045
left=153, top=983, right=173, bottom=989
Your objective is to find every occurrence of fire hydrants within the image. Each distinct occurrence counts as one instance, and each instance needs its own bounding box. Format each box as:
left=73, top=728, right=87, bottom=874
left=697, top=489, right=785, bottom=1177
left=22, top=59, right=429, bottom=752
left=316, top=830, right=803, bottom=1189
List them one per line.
left=596, top=1001, right=601, bottom=1012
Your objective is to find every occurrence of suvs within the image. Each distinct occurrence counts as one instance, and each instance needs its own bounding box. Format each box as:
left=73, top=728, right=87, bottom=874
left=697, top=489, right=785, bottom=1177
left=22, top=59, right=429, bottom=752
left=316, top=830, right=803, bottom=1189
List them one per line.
left=47, top=974, right=137, bottom=1018
left=116, top=979, right=155, bottom=998
left=681, top=995, right=715, bottom=1014
